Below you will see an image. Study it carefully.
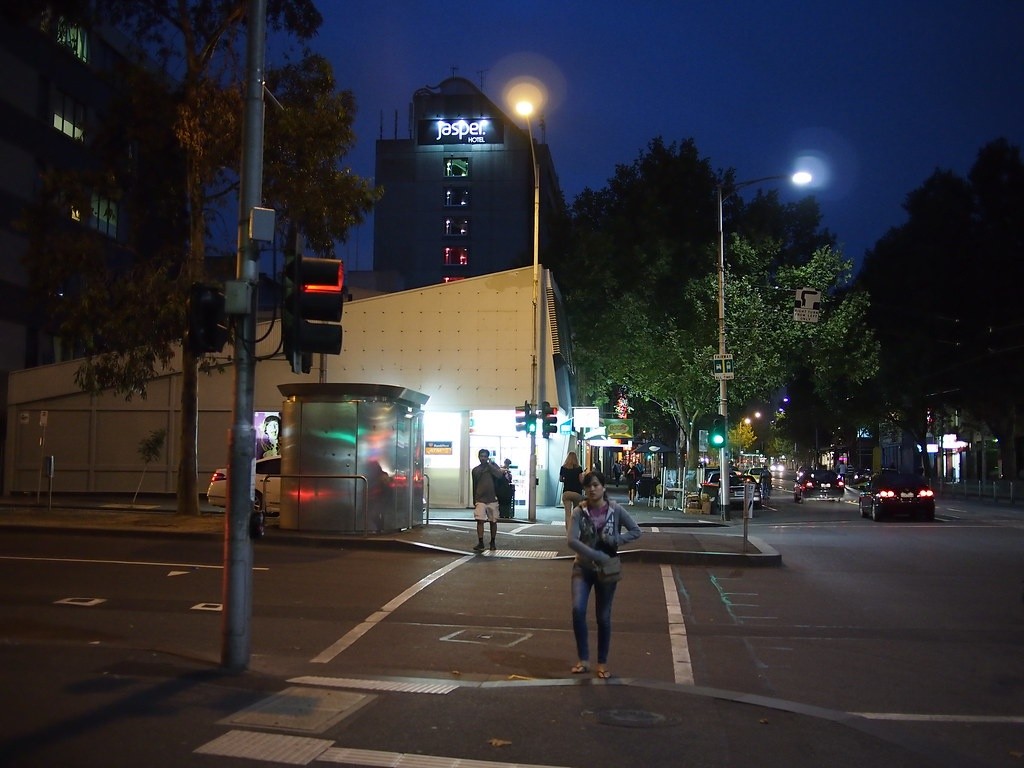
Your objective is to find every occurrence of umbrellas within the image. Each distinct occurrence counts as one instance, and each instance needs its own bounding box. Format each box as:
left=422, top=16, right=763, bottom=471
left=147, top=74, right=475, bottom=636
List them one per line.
left=634, top=441, right=673, bottom=479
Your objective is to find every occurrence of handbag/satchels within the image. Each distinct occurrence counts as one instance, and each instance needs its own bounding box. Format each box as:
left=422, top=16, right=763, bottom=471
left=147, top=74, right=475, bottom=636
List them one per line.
left=597, top=554, right=622, bottom=583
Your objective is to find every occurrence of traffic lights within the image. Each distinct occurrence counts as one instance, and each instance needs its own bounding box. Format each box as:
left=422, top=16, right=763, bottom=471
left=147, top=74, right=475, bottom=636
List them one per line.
left=528, top=414, right=536, bottom=435
left=289, top=232, right=345, bottom=376
left=178, top=282, right=227, bottom=355
left=515, top=401, right=529, bottom=432
left=543, top=401, right=558, bottom=439
left=709, top=413, right=726, bottom=448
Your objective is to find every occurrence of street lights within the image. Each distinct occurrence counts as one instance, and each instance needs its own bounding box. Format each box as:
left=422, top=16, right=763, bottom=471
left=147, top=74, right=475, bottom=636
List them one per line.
left=510, top=94, right=544, bottom=525
left=715, top=170, right=814, bottom=525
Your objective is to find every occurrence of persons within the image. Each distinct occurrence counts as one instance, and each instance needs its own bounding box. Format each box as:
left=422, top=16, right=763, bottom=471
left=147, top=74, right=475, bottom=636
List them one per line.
left=501, top=458, right=511, bottom=474
left=612, top=459, right=643, bottom=506
left=847, top=464, right=855, bottom=487
left=560, top=452, right=584, bottom=529
left=890, top=462, right=896, bottom=469
left=838, top=462, right=847, bottom=488
left=262, top=416, right=279, bottom=456
left=738, top=462, right=745, bottom=467
left=920, top=465, right=923, bottom=475
left=759, top=466, right=773, bottom=500
left=472, top=449, right=504, bottom=551
left=565, top=472, right=640, bottom=679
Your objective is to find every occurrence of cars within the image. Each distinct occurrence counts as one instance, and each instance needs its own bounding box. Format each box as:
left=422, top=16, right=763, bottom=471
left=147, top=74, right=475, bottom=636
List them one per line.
left=792, top=469, right=845, bottom=505
left=795, top=465, right=812, bottom=481
left=852, top=470, right=873, bottom=485
left=702, top=467, right=764, bottom=509
left=858, top=470, right=935, bottom=523
left=205, top=454, right=282, bottom=517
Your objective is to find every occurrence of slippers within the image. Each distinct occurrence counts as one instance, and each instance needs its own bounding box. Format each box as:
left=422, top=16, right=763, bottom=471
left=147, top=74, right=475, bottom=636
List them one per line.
left=572, top=665, right=589, bottom=674
left=598, top=670, right=611, bottom=679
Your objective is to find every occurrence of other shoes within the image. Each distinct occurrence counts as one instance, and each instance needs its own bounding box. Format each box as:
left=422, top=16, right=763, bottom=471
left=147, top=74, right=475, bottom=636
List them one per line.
left=473, top=543, right=484, bottom=550
left=490, top=542, right=496, bottom=551
left=628, top=500, right=634, bottom=506
